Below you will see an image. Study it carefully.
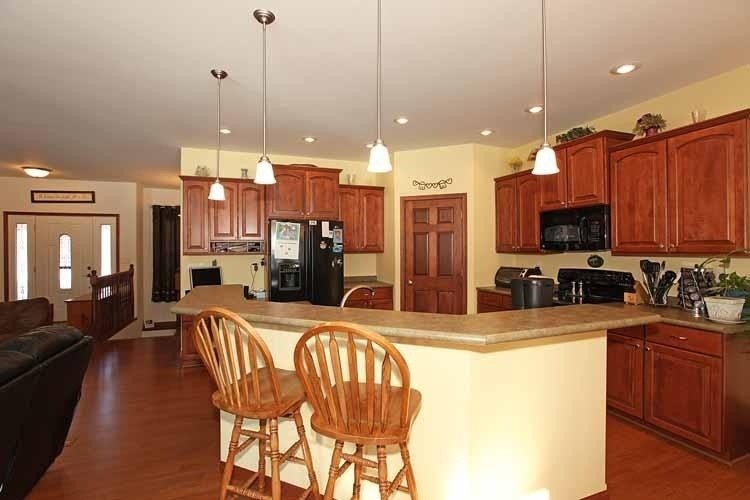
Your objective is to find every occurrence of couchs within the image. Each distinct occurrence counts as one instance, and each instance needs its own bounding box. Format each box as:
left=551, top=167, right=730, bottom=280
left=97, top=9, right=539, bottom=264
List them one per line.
left=0, top=297, right=94, bottom=399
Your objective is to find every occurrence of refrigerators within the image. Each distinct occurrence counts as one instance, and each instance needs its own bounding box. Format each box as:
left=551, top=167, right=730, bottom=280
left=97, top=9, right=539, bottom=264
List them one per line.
left=264, top=216, right=344, bottom=306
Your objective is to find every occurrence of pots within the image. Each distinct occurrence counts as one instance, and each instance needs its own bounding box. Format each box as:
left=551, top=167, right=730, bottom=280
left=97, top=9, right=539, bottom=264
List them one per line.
left=511, top=278, right=554, bottom=307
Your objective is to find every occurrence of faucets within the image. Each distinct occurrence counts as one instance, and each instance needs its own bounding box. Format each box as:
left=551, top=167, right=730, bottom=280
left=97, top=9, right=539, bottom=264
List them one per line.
left=339, top=285, right=376, bottom=308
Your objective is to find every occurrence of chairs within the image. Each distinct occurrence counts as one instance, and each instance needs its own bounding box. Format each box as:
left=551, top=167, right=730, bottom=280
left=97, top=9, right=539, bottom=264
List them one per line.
left=191, top=306, right=321, bottom=500
left=293, top=322, right=422, bottom=500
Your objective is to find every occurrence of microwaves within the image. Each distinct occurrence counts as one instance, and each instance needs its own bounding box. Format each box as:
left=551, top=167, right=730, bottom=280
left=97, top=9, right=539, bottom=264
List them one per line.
left=539, top=204, right=610, bottom=250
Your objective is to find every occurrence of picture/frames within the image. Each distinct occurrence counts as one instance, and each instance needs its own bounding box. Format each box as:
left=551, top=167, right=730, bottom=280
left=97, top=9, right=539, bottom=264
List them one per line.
left=30, top=190, right=95, bottom=203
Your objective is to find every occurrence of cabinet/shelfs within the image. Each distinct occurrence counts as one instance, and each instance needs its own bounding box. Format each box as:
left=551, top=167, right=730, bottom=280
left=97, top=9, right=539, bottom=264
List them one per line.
left=494, top=108, right=750, bottom=257
left=607, top=321, right=750, bottom=468
left=178, top=163, right=384, bottom=255
left=344, top=286, right=393, bottom=310
left=476, top=288, right=512, bottom=313
left=64, top=288, right=120, bottom=335
left=181, top=314, right=210, bottom=369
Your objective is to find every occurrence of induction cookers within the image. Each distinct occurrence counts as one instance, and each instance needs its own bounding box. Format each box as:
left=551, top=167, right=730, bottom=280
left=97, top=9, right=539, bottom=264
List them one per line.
left=554, top=284, right=634, bottom=305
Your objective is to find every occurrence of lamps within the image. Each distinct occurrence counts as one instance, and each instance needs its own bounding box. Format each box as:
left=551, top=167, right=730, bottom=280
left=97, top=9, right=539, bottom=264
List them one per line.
left=531, top=0, right=561, bottom=176
left=208, top=69, right=228, bottom=203
left=367, top=0, right=393, bottom=175
left=252, top=9, right=277, bottom=185
left=21, top=167, right=52, bottom=178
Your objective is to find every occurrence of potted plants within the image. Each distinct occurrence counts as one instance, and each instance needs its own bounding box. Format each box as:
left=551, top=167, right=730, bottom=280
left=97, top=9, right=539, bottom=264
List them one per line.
left=697, top=248, right=750, bottom=324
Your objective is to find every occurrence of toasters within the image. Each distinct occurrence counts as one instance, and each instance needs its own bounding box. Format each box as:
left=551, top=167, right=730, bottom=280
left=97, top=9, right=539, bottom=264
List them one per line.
left=495, top=266, right=541, bottom=289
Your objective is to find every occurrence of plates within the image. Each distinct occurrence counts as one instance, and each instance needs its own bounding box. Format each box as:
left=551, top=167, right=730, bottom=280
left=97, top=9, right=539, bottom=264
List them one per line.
left=706, top=318, right=747, bottom=324
